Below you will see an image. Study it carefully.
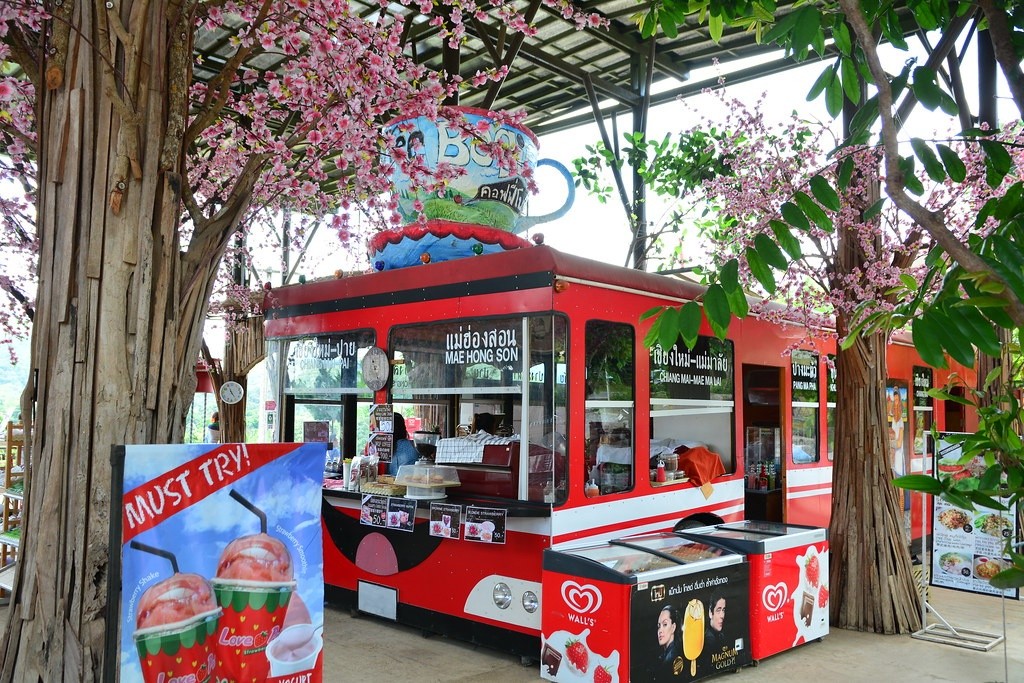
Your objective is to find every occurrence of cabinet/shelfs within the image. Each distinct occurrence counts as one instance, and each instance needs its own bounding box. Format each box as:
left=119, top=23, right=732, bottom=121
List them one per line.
left=0, top=421, right=44, bottom=599
left=746, top=427, right=775, bottom=471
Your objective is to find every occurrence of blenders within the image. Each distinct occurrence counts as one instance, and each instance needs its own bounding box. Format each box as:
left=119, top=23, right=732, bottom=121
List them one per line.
left=413, top=431, right=441, bottom=465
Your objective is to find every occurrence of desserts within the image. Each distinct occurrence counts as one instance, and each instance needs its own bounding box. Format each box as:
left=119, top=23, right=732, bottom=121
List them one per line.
left=404, top=473, right=443, bottom=484
left=363, top=475, right=406, bottom=496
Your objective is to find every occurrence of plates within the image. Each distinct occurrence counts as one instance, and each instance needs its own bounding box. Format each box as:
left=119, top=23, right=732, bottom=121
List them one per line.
left=938, top=509, right=973, bottom=530
left=939, top=552, right=972, bottom=574
left=973, top=513, right=1014, bottom=539
left=973, top=557, right=1012, bottom=579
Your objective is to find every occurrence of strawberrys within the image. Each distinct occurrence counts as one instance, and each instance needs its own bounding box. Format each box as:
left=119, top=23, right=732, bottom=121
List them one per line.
left=818, top=584, right=829, bottom=608
left=805, top=555, right=819, bottom=589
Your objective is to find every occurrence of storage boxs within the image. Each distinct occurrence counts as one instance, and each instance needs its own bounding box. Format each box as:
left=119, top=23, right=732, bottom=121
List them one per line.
left=394, top=466, right=461, bottom=488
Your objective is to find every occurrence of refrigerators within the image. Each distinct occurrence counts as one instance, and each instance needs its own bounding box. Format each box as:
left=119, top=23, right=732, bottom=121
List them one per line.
left=678, top=519, right=829, bottom=667
left=541, top=532, right=753, bottom=683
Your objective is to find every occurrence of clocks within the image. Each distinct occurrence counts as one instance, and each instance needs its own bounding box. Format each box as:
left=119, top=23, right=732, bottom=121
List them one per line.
left=221, top=382, right=245, bottom=404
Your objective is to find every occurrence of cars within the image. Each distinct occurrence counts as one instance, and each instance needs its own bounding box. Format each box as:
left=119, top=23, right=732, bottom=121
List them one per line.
left=743, top=439, right=812, bottom=472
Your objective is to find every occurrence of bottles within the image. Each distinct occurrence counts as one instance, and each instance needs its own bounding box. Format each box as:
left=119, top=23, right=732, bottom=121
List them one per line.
left=342, top=458, right=351, bottom=487
left=748, top=461, right=775, bottom=492
left=588, top=479, right=599, bottom=496
left=657, top=460, right=665, bottom=482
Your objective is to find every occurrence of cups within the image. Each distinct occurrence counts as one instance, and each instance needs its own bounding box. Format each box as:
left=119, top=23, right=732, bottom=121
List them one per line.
left=211, top=578, right=295, bottom=683
left=132, top=605, right=221, bottom=683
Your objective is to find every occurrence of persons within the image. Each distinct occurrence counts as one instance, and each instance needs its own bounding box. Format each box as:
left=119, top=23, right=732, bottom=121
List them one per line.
left=700, top=589, right=745, bottom=677
left=653, top=604, right=689, bottom=683
left=208, top=411, right=221, bottom=443
left=387, top=412, right=420, bottom=477
